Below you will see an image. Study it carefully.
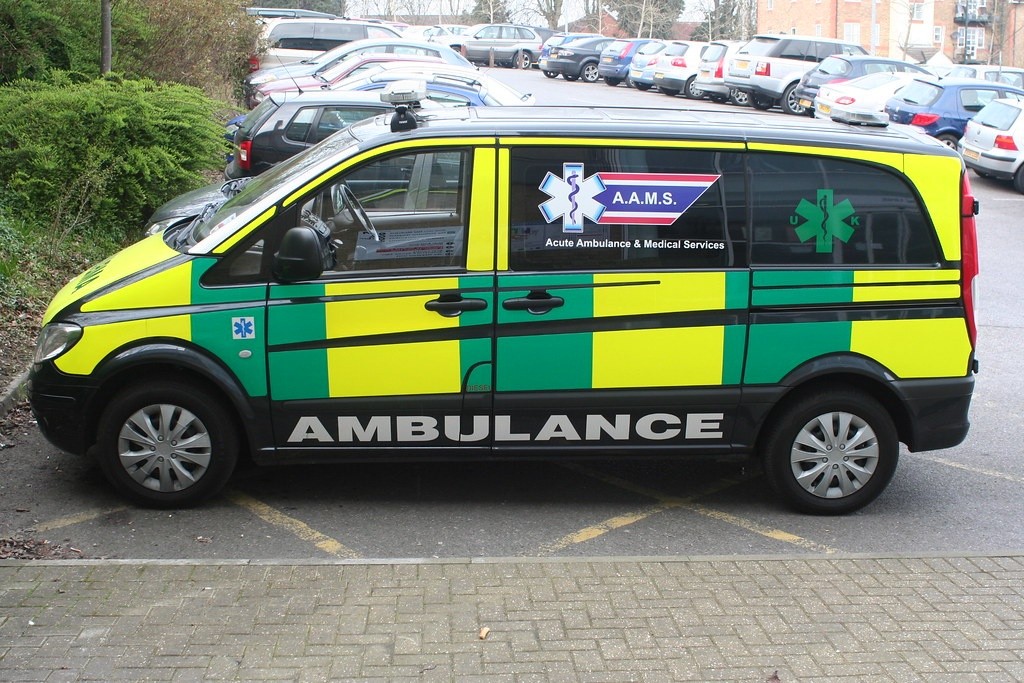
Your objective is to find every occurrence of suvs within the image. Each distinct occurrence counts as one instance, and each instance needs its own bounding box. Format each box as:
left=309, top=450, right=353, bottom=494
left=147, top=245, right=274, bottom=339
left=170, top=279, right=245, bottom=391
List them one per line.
left=458, top=24, right=542, bottom=69
left=223, top=53, right=467, bottom=213
left=654, top=40, right=718, bottom=101
left=598, top=39, right=667, bottom=88
left=957, top=92, right=1024, bottom=195
left=732, top=34, right=871, bottom=117
left=883, top=70, right=1024, bottom=151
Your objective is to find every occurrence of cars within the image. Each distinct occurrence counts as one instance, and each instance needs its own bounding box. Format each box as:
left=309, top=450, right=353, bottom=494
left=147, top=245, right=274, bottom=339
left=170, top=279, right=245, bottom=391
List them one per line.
left=631, top=43, right=671, bottom=90
left=221, top=37, right=532, bottom=158
left=393, top=23, right=469, bottom=43
left=795, top=54, right=934, bottom=124
left=941, top=65, right=1024, bottom=99
left=814, top=66, right=918, bottom=126
left=537, top=32, right=619, bottom=83
left=142, top=151, right=468, bottom=270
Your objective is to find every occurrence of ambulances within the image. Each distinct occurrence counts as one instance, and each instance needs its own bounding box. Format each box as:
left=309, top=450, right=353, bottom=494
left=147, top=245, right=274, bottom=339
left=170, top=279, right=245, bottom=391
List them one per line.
left=25, top=77, right=978, bottom=514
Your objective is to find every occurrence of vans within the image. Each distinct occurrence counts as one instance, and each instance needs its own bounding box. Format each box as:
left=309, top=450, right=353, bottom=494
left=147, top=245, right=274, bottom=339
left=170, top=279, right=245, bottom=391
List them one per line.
left=695, top=40, right=749, bottom=106
left=242, top=7, right=405, bottom=73
